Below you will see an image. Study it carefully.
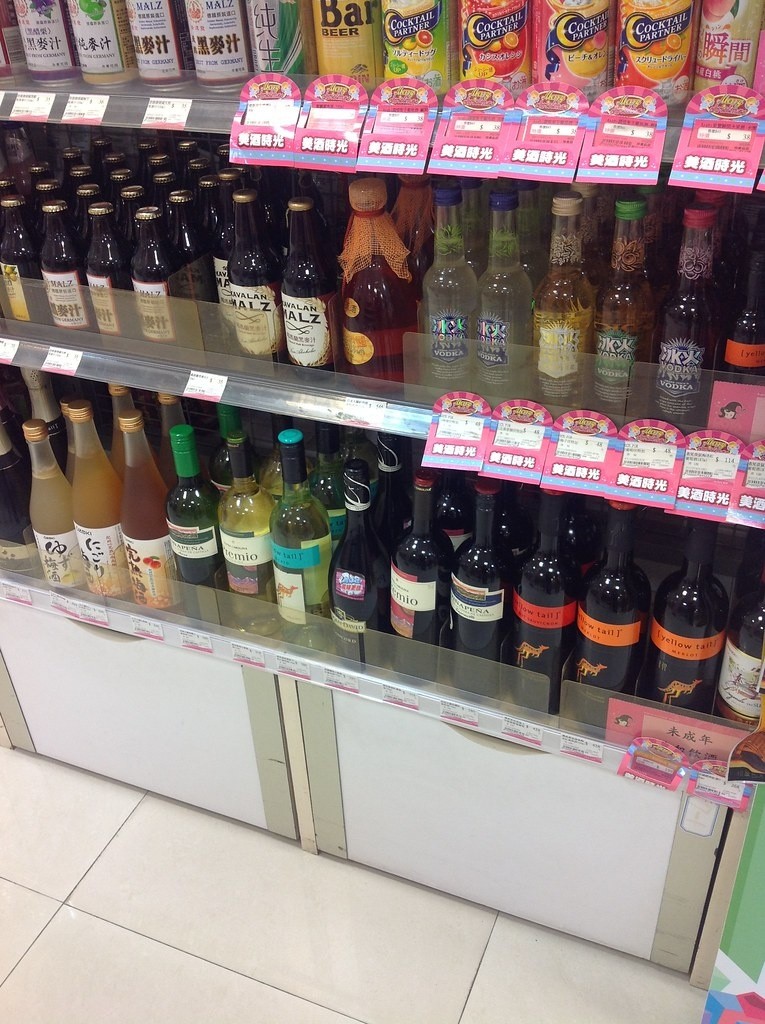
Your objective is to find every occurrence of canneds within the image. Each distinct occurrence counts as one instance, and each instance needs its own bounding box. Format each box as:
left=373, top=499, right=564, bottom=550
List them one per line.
left=0, top=0, right=765, bottom=123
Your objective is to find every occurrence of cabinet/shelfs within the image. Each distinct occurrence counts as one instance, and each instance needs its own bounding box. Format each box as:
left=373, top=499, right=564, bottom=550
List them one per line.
left=0, top=0, right=765, bottom=990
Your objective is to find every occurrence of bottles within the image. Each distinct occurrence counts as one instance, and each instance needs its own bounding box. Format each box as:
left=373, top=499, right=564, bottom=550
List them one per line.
left=0, top=123, right=765, bottom=730
